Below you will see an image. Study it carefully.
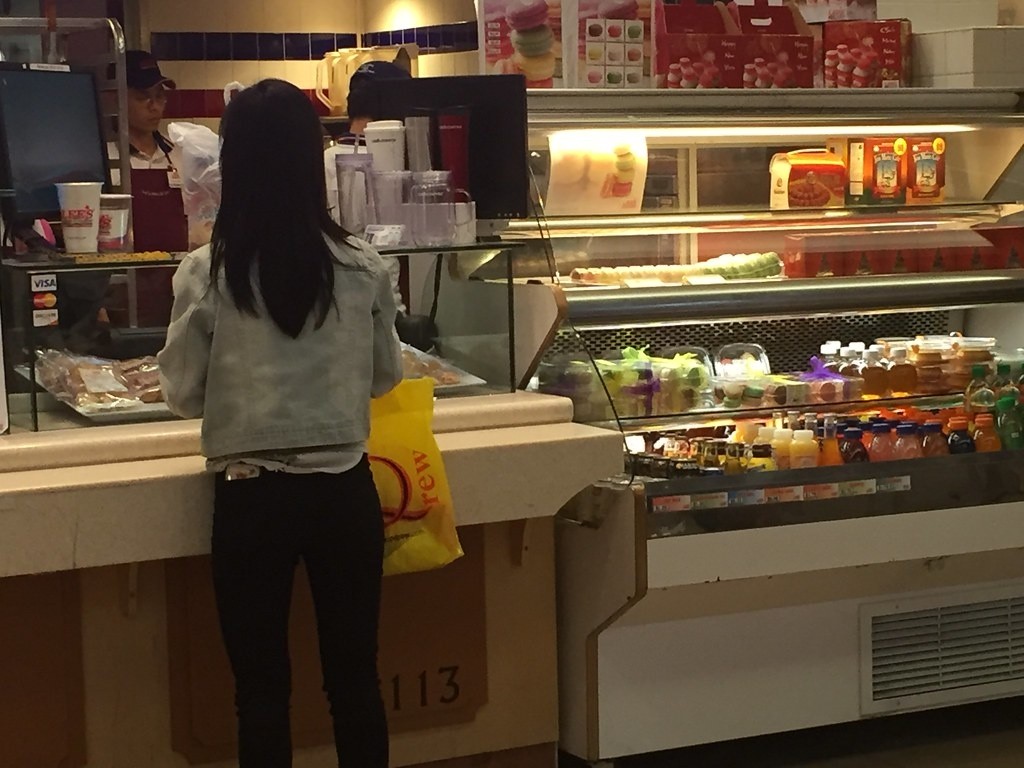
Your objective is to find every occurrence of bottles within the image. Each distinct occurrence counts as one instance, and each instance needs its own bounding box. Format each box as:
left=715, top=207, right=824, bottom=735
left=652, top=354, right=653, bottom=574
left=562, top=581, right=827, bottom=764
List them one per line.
left=821, top=337, right=912, bottom=396
left=631, top=363, right=1024, bottom=478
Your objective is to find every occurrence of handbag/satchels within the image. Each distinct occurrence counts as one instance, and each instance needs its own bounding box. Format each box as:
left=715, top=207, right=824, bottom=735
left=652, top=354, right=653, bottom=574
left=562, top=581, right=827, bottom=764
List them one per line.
left=361, top=375, right=463, bottom=579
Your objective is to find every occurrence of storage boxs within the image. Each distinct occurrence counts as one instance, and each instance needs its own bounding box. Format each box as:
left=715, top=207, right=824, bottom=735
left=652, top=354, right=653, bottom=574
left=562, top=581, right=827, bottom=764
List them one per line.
left=652, top=0, right=946, bottom=212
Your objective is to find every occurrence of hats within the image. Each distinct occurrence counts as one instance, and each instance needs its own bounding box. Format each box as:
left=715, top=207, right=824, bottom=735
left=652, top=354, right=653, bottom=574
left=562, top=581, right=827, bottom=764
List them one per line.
left=106, top=50, right=177, bottom=90
left=348, top=59, right=411, bottom=100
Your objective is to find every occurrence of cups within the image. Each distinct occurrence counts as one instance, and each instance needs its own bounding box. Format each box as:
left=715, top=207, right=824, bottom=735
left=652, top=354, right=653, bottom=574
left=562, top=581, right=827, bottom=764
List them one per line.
left=57, top=182, right=134, bottom=253
left=335, top=119, right=474, bottom=249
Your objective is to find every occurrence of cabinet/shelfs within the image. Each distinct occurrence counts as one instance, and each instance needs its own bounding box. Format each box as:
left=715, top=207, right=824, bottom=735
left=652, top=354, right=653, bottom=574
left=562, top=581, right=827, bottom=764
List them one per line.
left=399, top=84, right=1024, bottom=768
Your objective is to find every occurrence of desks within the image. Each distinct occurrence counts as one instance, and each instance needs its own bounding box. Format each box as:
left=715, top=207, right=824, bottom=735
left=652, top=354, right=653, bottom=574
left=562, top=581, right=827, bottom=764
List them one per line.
left=0, top=348, right=624, bottom=768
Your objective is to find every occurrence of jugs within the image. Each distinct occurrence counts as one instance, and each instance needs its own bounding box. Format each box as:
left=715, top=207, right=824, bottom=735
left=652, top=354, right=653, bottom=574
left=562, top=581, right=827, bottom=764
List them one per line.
left=317, top=45, right=402, bottom=116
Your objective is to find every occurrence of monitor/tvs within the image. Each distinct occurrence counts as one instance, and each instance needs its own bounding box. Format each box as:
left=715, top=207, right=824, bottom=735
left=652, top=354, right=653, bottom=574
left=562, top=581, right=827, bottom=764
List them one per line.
left=371, top=73, right=531, bottom=219
left=0, top=60, right=114, bottom=229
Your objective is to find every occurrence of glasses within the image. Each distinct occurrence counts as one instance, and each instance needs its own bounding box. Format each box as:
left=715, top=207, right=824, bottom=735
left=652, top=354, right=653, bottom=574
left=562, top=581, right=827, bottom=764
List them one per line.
left=126, top=88, right=168, bottom=102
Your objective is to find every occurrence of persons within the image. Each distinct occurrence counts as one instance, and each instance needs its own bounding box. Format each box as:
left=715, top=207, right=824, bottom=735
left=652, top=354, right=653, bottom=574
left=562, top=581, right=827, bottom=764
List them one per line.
left=107, top=50, right=189, bottom=328
left=156, top=77, right=405, bottom=768
left=323, top=60, right=412, bottom=227
